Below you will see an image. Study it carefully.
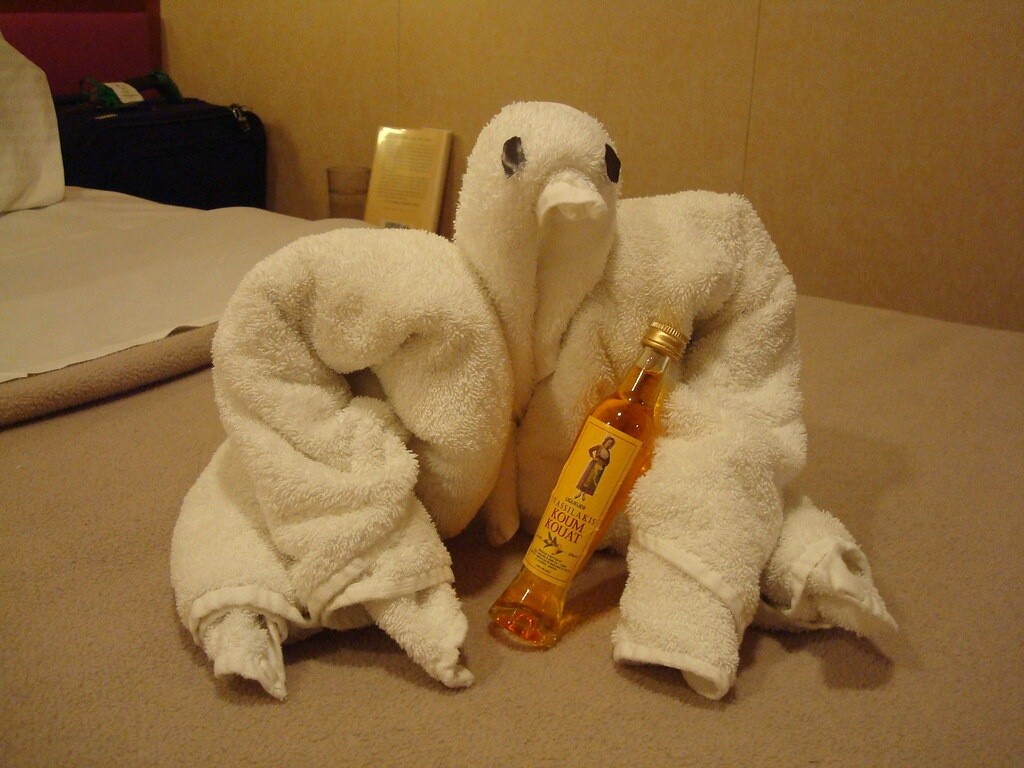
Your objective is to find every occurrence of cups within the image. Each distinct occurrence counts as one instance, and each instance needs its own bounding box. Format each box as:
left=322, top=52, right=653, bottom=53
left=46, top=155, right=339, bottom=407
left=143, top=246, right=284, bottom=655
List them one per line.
left=327, top=166, right=371, bottom=221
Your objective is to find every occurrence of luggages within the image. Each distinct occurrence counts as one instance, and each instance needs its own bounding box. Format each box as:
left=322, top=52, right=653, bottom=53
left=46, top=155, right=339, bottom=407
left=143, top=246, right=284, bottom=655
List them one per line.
left=57, top=69, right=269, bottom=211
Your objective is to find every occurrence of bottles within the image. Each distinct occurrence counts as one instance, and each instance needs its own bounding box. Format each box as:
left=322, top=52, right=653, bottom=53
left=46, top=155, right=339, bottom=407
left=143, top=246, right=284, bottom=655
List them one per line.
left=490, top=322, right=688, bottom=648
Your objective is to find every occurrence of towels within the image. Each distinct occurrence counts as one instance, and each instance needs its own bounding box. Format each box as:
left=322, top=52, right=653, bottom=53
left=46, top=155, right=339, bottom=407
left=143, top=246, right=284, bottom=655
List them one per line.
left=165, top=99, right=902, bottom=706
